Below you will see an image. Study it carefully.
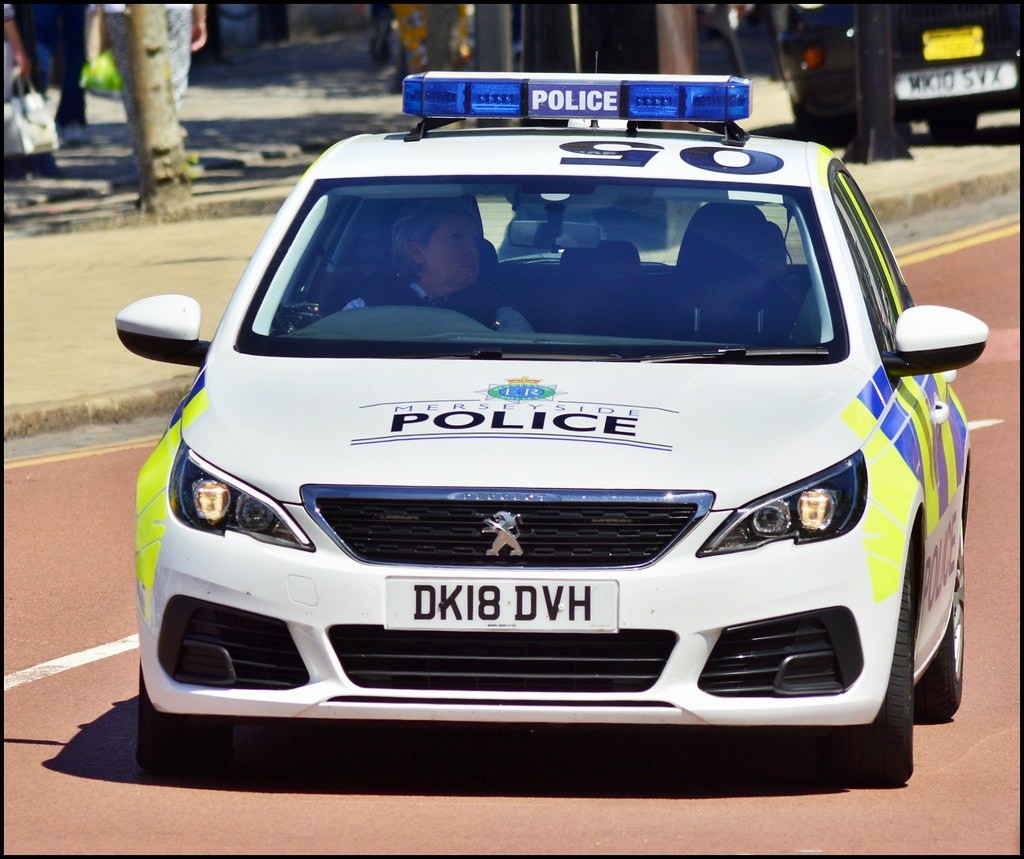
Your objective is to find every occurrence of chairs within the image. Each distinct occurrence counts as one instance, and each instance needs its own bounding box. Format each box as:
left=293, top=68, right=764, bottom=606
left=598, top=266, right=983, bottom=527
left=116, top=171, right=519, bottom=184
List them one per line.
left=663, top=201, right=792, bottom=343
left=555, top=240, right=651, bottom=335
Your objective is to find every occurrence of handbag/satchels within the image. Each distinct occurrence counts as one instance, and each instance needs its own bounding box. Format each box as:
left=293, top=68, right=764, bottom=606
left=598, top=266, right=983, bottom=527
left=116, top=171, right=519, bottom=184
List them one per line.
left=3, top=65, right=61, bottom=157
left=79, top=48, right=123, bottom=98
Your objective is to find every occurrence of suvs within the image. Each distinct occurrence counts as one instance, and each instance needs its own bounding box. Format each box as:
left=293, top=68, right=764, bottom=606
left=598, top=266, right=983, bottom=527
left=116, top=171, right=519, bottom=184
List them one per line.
left=781, top=0, right=1024, bottom=139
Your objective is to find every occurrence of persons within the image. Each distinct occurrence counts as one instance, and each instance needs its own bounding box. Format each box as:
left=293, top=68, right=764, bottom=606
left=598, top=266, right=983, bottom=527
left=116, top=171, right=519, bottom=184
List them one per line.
left=342, top=201, right=534, bottom=335
left=4, top=4, right=207, bottom=158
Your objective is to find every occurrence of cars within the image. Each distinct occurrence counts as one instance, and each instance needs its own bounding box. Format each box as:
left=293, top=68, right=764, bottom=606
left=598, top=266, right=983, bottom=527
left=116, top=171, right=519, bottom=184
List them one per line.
left=112, top=71, right=989, bottom=787
